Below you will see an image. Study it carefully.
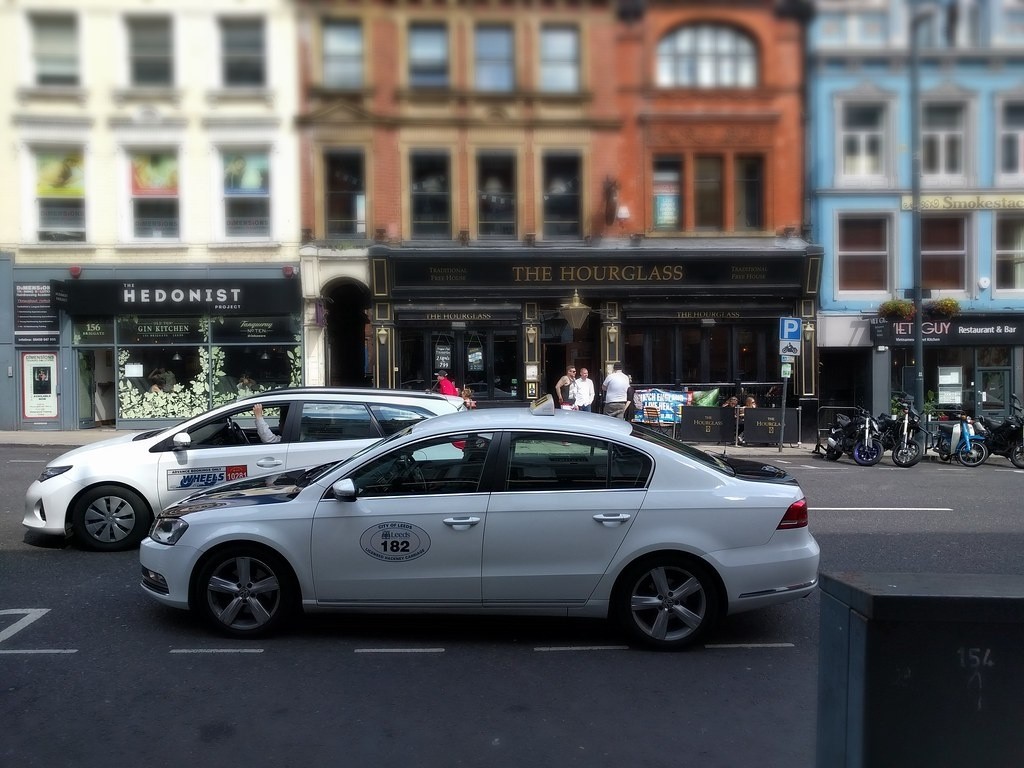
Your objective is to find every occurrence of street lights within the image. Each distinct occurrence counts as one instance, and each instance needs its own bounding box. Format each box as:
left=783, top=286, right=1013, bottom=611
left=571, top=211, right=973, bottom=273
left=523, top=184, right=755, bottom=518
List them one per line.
left=904, top=7, right=936, bottom=444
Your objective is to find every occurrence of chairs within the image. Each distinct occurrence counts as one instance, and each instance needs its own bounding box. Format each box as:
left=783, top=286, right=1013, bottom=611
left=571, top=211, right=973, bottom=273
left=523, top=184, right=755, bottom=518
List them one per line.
left=646, top=407, right=673, bottom=437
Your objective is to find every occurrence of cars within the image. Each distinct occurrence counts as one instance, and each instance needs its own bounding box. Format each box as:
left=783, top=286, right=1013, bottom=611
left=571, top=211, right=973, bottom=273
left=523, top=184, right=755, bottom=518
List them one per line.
left=18, top=384, right=472, bottom=554
left=137, top=412, right=824, bottom=651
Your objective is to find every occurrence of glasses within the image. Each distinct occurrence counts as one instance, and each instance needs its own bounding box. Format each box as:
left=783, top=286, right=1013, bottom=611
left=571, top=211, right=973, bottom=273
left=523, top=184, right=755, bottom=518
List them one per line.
left=570, top=371, right=576, bottom=374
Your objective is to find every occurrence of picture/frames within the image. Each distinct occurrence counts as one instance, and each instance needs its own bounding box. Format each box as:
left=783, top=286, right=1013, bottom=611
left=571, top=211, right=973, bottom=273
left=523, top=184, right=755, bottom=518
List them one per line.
left=106, top=351, right=113, bottom=367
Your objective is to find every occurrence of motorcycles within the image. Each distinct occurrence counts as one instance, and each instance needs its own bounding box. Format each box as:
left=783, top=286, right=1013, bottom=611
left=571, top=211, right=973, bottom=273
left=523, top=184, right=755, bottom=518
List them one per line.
left=824, top=405, right=885, bottom=467
left=979, top=392, right=1024, bottom=469
left=873, top=390, right=925, bottom=468
left=932, top=406, right=988, bottom=468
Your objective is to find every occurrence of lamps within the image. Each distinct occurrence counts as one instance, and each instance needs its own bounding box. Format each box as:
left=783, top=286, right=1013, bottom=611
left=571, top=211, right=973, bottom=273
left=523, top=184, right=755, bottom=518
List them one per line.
left=525, top=233, right=536, bottom=241
left=260, top=347, right=270, bottom=359
left=784, top=227, right=795, bottom=236
left=527, top=321, right=537, bottom=343
left=618, top=206, right=628, bottom=225
left=609, top=322, right=617, bottom=342
left=630, top=233, right=645, bottom=241
left=172, top=351, right=181, bottom=360
left=378, top=325, right=388, bottom=344
left=460, top=231, right=469, bottom=240
left=376, top=228, right=386, bottom=237
left=560, top=289, right=592, bottom=329
left=701, top=319, right=717, bottom=324
left=302, top=229, right=314, bottom=241
left=451, top=322, right=467, bottom=328
left=803, top=321, right=815, bottom=340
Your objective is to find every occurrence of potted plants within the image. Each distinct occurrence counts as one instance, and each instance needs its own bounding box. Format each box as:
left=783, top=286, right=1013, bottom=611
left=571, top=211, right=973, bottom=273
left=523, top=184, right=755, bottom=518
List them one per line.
left=890, top=396, right=899, bottom=420
left=929, top=301, right=957, bottom=320
left=879, top=303, right=917, bottom=321
left=922, top=390, right=936, bottom=421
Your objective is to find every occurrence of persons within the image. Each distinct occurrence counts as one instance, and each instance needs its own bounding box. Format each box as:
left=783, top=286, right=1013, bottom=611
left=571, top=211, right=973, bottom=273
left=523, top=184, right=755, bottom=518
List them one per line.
left=148, top=367, right=176, bottom=393
left=722, top=396, right=738, bottom=407
left=601, top=362, right=635, bottom=422
left=555, top=365, right=577, bottom=446
left=449, top=379, right=476, bottom=408
left=745, top=397, right=756, bottom=408
left=434, top=370, right=458, bottom=396
left=575, top=368, right=595, bottom=412
left=253, top=403, right=282, bottom=443
left=237, top=375, right=256, bottom=391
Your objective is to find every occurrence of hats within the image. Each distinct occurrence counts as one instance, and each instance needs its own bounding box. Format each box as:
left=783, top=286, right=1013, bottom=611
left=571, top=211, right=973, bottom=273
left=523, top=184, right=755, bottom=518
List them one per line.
left=434, top=370, right=448, bottom=377
left=614, top=362, right=622, bottom=370
